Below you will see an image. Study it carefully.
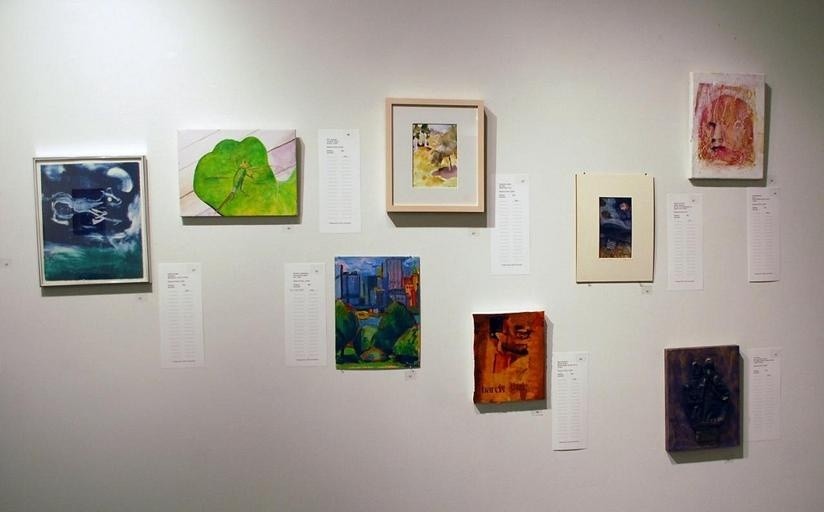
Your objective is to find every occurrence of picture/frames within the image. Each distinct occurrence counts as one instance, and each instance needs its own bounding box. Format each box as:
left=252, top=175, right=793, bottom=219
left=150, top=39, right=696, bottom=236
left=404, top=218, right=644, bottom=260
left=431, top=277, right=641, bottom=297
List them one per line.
left=385, top=98, right=485, bottom=212
left=33, top=156, right=152, bottom=287
